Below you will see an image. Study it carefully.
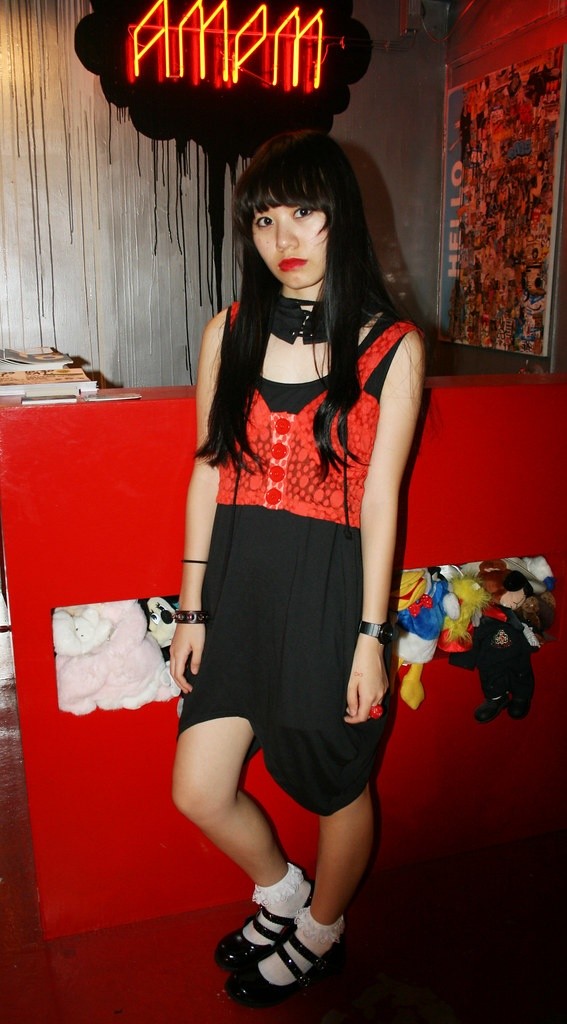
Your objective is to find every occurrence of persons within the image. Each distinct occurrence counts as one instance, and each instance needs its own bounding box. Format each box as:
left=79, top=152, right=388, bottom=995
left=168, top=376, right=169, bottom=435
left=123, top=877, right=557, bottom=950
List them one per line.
left=167, top=128, right=428, bottom=1009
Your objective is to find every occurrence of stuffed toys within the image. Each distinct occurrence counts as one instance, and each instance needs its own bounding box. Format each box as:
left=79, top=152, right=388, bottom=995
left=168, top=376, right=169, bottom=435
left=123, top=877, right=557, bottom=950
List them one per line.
left=397, top=556, right=555, bottom=722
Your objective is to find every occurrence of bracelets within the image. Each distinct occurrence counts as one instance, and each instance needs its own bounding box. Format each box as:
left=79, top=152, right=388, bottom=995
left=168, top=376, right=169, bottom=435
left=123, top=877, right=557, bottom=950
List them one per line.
left=183, top=560, right=208, bottom=564
left=174, top=609, right=209, bottom=624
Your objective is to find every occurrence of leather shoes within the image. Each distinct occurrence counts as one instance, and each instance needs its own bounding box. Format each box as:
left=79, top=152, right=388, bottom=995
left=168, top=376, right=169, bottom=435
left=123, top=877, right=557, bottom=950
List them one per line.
left=224, top=932, right=347, bottom=1007
left=214, top=880, right=314, bottom=969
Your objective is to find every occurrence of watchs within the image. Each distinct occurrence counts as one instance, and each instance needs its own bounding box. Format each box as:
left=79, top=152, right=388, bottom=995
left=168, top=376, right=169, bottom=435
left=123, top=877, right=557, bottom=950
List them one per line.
left=357, top=620, right=399, bottom=645
